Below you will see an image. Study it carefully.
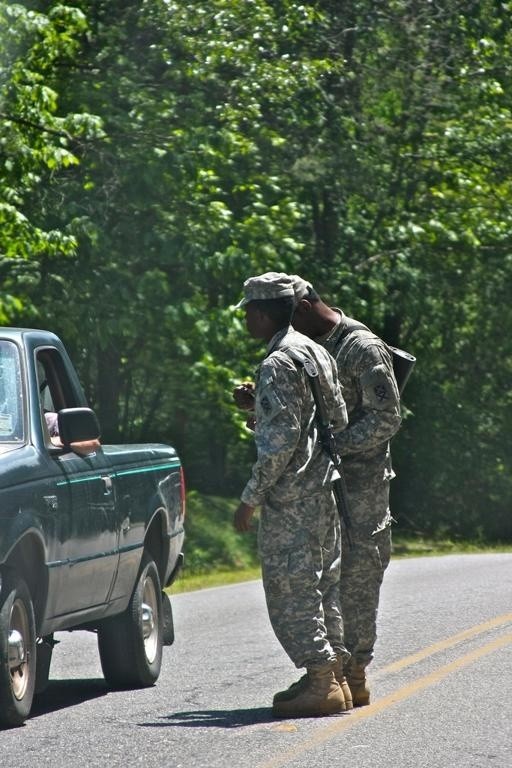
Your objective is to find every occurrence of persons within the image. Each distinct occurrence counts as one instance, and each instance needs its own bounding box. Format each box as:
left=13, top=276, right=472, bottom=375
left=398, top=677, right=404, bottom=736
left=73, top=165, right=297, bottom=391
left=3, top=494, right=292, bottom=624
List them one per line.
left=233, top=275, right=401, bottom=705
left=231, top=271, right=354, bottom=717
left=42, top=407, right=101, bottom=456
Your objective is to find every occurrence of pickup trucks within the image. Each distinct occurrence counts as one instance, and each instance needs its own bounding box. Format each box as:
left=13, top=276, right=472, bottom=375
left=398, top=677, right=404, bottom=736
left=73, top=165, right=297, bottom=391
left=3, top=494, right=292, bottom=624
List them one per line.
left=0, top=327, right=186, bottom=728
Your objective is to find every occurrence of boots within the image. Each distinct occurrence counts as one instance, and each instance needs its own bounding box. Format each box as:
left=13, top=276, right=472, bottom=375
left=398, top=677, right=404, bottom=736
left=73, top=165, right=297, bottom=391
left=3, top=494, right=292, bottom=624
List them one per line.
left=271, top=656, right=371, bottom=716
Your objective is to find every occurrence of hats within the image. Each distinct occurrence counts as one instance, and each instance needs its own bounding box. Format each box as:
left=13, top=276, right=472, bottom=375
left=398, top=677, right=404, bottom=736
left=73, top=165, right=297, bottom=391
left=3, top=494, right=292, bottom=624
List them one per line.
left=235, top=271, right=313, bottom=323
